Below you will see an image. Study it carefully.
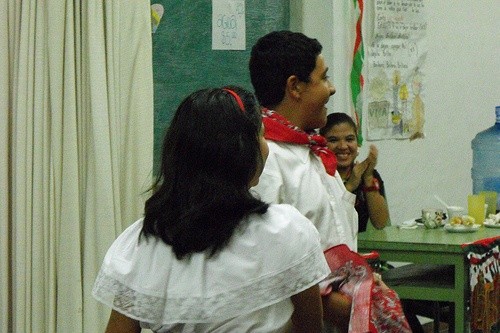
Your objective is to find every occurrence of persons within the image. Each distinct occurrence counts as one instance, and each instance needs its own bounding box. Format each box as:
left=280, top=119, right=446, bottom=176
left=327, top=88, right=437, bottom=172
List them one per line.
left=92, top=84, right=331, bottom=333
left=248, top=30, right=413, bottom=333
left=320, top=112, right=425, bottom=333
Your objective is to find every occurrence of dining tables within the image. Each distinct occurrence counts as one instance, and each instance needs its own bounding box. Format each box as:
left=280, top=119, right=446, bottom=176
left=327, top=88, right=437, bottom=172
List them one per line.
left=356, top=225, right=500, bottom=333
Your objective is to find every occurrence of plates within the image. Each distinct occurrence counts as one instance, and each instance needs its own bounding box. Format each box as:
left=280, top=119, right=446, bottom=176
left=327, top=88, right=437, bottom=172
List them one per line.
left=482, top=222, right=500, bottom=228
left=445, top=224, right=482, bottom=233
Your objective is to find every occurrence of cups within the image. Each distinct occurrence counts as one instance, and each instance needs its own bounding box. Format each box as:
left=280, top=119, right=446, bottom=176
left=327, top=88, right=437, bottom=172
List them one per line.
left=421, top=208, right=443, bottom=229
left=480, top=191, right=497, bottom=217
left=468, top=194, right=485, bottom=225
left=446, top=204, right=463, bottom=220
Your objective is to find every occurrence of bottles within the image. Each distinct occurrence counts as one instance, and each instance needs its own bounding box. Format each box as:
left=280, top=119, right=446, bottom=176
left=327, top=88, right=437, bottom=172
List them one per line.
left=470, top=106, right=500, bottom=211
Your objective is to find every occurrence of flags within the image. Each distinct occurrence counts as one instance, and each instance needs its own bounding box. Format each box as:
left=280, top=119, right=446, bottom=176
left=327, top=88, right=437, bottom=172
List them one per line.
left=350, top=0, right=364, bottom=147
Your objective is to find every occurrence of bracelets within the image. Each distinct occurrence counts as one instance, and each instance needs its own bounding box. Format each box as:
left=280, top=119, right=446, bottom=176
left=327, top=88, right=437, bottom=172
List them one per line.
left=364, top=178, right=379, bottom=192
